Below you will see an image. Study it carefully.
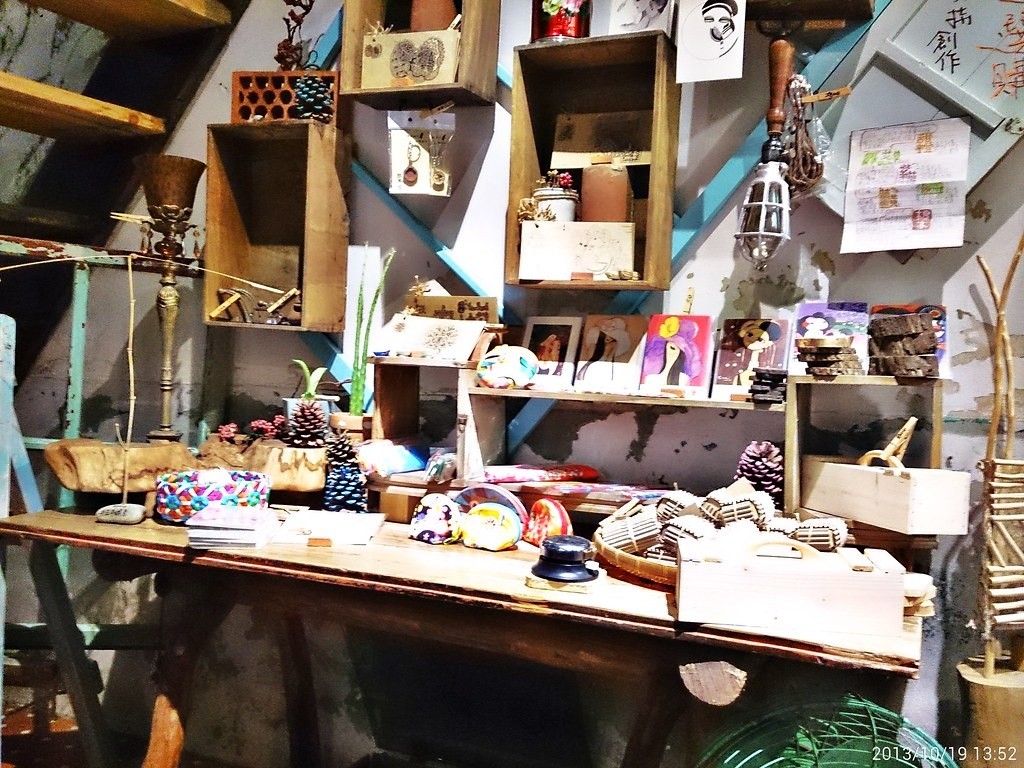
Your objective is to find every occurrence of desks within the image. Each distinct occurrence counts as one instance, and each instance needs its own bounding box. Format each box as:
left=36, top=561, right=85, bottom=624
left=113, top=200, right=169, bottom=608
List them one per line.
left=0, top=485, right=931, bottom=768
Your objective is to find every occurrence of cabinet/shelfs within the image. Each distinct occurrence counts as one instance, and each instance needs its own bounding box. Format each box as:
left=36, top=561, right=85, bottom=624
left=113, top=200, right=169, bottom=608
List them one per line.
left=362, top=351, right=943, bottom=552
left=339, top=0, right=502, bottom=111
left=201, top=118, right=347, bottom=334
left=0, top=235, right=216, bottom=652
left=504, top=30, right=684, bottom=292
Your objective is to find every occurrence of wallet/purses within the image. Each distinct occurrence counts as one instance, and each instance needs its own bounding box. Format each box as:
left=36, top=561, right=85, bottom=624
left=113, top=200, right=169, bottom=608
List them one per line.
left=463, top=503, right=522, bottom=552
left=408, top=494, right=463, bottom=545
left=524, top=498, right=573, bottom=545
left=455, top=483, right=530, bottom=543
